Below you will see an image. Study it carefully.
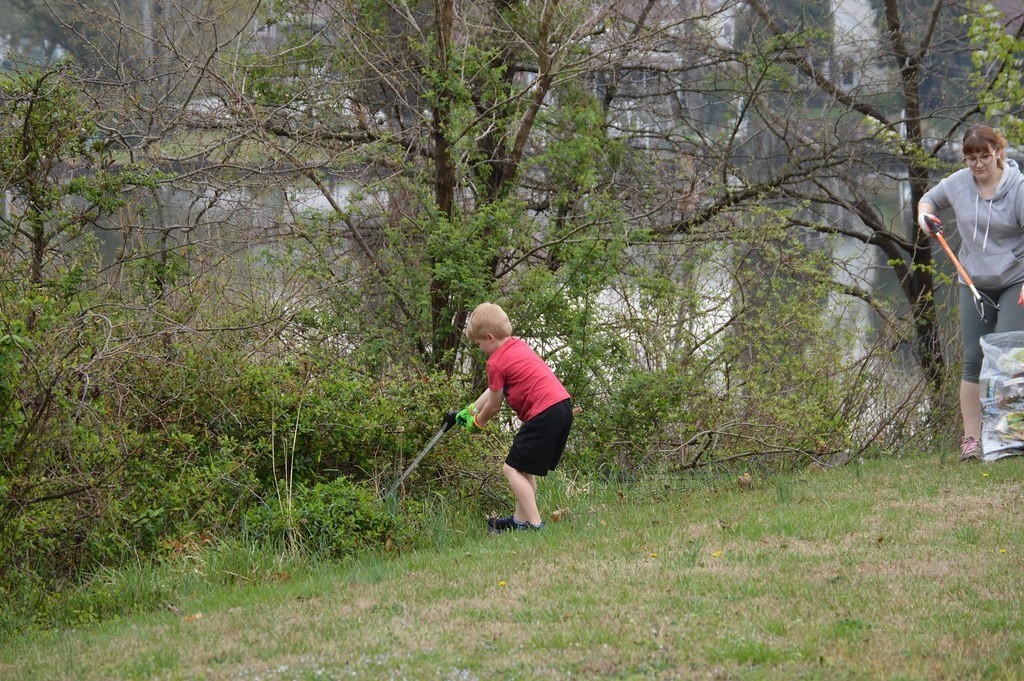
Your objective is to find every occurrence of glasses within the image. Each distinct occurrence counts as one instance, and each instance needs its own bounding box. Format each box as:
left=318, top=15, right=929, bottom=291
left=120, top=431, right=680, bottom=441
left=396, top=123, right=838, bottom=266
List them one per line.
left=963, top=147, right=996, bottom=167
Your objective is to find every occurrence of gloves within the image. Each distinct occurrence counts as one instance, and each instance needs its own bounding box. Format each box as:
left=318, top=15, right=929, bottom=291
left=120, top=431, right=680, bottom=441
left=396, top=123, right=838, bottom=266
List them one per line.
left=455, top=406, right=479, bottom=426
left=919, top=213, right=945, bottom=238
left=1017, top=284, right=1024, bottom=306
left=466, top=416, right=484, bottom=435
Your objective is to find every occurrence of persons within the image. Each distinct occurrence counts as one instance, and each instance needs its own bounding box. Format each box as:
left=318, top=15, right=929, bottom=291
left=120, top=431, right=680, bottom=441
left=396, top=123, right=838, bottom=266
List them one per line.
left=456, top=301, right=573, bottom=533
left=917, top=125, right=1024, bottom=462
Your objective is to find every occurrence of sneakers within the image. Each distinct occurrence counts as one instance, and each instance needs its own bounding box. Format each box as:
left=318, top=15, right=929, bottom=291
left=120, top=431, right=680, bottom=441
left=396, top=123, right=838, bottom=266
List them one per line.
left=522, top=521, right=544, bottom=531
left=959, top=435, right=982, bottom=463
left=486, top=515, right=522, bottom=533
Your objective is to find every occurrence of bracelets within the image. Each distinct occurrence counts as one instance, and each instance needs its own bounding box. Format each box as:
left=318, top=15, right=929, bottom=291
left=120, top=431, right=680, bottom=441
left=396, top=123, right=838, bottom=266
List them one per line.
left=470, top=404, right=479, bottom=414
